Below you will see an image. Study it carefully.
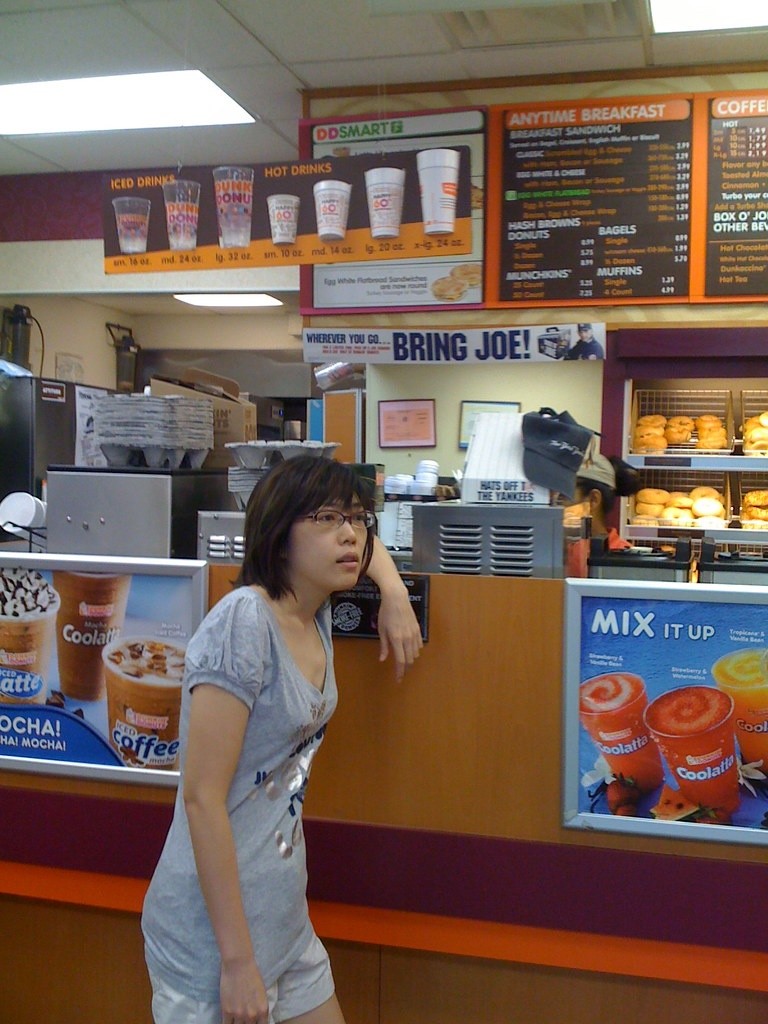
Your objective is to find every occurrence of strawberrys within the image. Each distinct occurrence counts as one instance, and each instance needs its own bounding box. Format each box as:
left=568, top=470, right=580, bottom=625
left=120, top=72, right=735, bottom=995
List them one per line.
left=693, top=804, right=732, bottom=825
left=605, top=773, right=642, bottom=817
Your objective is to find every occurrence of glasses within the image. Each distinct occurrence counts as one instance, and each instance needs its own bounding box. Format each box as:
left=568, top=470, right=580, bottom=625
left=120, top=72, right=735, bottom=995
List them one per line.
left=298, top=509, right=377, bottom=530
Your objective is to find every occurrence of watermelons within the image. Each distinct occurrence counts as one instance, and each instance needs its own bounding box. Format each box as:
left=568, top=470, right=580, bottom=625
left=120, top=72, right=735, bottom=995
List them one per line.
left=650, top=784, right=701, bottom=822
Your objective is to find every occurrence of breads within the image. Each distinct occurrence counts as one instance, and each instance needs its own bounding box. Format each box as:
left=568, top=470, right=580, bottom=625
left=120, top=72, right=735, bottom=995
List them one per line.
left=741, top=488, right=768, bottom=530
left=742, top=410, right=768, bottom=455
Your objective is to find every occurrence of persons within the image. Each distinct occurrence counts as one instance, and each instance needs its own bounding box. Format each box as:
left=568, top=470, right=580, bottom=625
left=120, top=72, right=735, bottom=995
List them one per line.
left=563, top=323, right=604, bottom=360
left=553, top=453, right=645, bottom=577
left=140, top=458, right=424, bottom=1024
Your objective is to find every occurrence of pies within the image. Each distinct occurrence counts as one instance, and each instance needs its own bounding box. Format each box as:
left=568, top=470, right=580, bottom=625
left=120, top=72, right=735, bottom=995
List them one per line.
left=432, top=263, right=481, bottom=302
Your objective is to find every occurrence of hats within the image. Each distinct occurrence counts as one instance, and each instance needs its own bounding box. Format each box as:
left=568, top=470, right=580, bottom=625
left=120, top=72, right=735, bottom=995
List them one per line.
left=576, top=451, right=616, bottom=488
left=522, top=407, right=592, bottom=497
left=578, top=323, right=591, bottom=333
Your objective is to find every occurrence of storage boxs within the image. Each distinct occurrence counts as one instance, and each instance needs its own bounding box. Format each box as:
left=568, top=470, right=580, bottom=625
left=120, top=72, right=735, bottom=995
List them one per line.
left=151, top=359, right=257, bottom=469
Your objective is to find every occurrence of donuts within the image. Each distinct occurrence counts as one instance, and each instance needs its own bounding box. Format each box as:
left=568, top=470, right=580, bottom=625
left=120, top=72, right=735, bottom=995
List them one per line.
left=631, top=414, right=728, bottom=453
left=631, top=486, right=729, bottom=530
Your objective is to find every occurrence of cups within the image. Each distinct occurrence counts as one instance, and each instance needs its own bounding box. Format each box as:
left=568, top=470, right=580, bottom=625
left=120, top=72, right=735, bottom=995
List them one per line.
left=53, top=568, right=132, bottom=702
left=363, top=167, right=406, bottom=237
left=0, top=585, right=61, bottom=706
left=579, top=672, right=664, bottom=793
left=711, top=647, right=768, bottom=778
left=313, top=359, right=354, bottom=391
left=642, top=686, right=741, bottom=812
left=162, top=180, right=201, bottom=251
left=416, top=149, right=460, bottom=235
left=213, top=166, right=255, bottom=247
left=103, top=635, right=186, bottom=770
left=265, top=194, right=300, bottom=243
left=0, top=492, right=48, bottom=553
left=113, top=197, right=152, bottom=253
left=312, top=180, right=351, bottom=237
